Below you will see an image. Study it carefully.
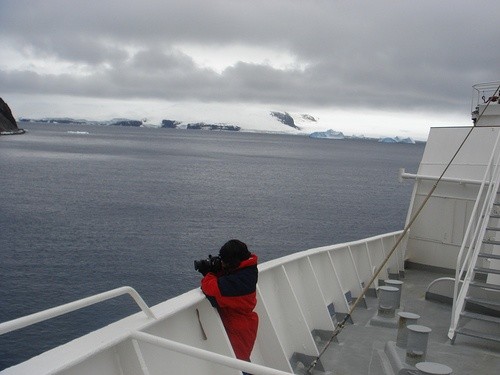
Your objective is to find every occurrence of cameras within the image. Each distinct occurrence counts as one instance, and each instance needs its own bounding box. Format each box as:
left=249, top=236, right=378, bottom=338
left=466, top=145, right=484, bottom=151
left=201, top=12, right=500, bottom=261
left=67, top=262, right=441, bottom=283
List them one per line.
left=194, top=255, right=222, bottom=275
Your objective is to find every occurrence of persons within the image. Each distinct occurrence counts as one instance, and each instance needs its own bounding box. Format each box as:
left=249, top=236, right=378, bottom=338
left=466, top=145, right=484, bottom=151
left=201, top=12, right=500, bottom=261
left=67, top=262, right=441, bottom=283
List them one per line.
left=200, top=239, right=259, bottom=375
